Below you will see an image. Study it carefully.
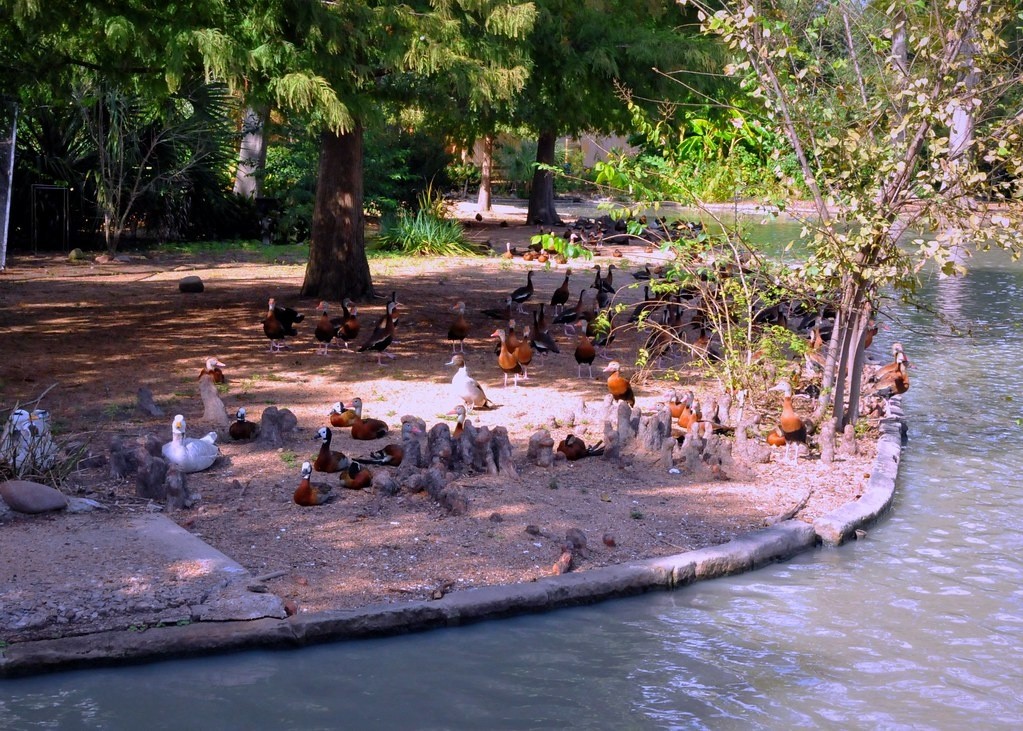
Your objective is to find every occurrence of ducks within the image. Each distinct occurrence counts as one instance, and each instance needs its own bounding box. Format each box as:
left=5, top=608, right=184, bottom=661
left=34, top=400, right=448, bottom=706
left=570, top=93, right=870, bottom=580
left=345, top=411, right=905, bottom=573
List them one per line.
left=314, top=301, right=334, bottom=356
left=491, top=328, right=523, bottom=388
left=480, top=296, right=512, bottom=321
left=448, top=301, right=469, bottom=353
left=529, top=311, right=560, bottom=365
left=357, top=291, right=400, bottom=367
left=160, top=414, right=218, bottom=473
left=198, top=356, right=226, bottom=382
left=474, top=211, right=734, bottom=264
left=346, top=398, right=389, bottom=440
left=445, top=405, right=467, bottom=438
left=314, top=427, right=349, bottom=473
left=588, top=262, right=919, bottom=446
left=557, top=434, right=605, bottom=460
left=263, top=298, right=285, bottom=353
left=512, top=271, right=536, bottom=315
left=536, top=303, right=548, bottom=335
left=550, top=269, right=573, bottom=317
left=574, top=319, right=596, bottom=379
left=494, top=319, right=520, bottom=356
left=293, top=461, right=333, bottom=506
left=513, top=326, right=534, bottom=380
left=375, top=444, right=403, bottom=466
left=0, top=410, right=60, bottom=472
left=273, top=307, right=305, bottom=347
left=229, top=407, right=258, bottom=440
left=327, top=402, right=355, bottom=427
left=552, top=289, right=587, bottom=336
left=331, top=299, right=353, bottom=348
left=339, top=461, right=372, bottom=490
left=444, top=354, right=492, bottom=412
left=336, top=306, right=361, bottom=350
